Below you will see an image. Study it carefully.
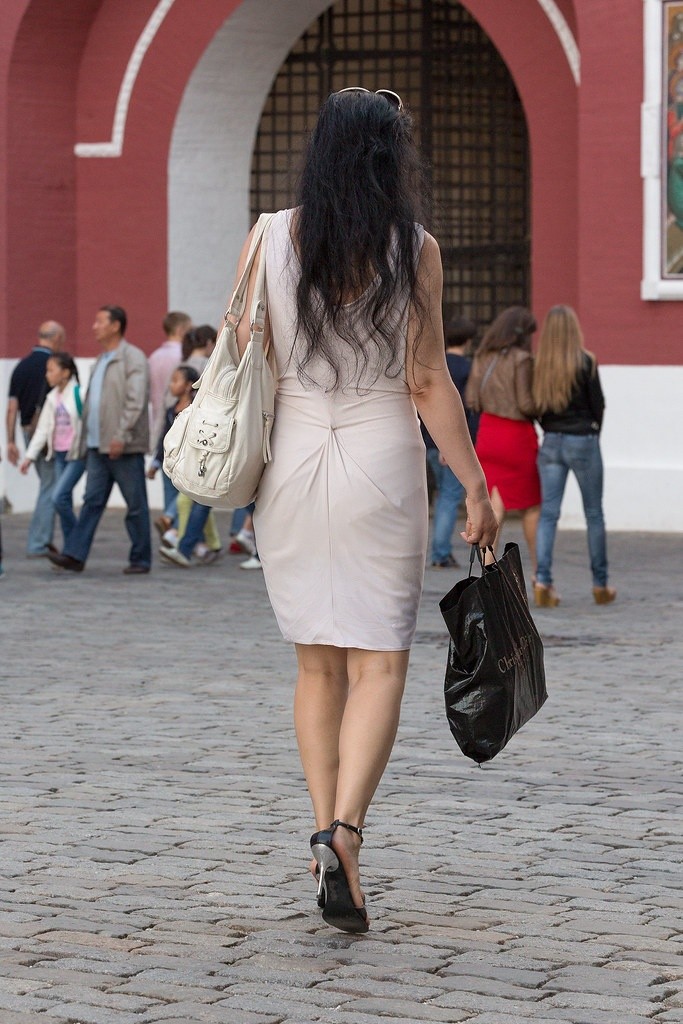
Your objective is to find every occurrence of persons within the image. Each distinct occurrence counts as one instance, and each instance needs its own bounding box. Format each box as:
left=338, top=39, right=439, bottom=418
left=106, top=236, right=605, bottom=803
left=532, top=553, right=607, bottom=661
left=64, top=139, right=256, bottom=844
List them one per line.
left=217, top=86, right=497, bottom=933
left=531, top=306, right=617, bottom=607
left=148, top=312, right=262, bottom=570
left=4, top=320, right=66, bottom=558
left=421, top=316, right=484, bottom=568
left=21, top=356, right=86, bottom=543
left=48, top=304, right=150, bottom=574
left=466, top=305, right=543, bottom=567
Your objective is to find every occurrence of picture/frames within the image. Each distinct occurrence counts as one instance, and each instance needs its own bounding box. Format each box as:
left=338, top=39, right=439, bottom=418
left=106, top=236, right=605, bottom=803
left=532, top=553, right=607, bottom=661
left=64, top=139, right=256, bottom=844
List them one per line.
left=641, top=0, right=683, bottom=301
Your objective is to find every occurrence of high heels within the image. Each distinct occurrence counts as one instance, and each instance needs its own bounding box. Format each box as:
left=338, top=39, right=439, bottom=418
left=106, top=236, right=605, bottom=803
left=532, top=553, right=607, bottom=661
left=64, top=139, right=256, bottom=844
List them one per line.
left=310, top=819, right=370, bottom=934
left=531, top=584, right=561, bottom=609
left=591, top=586, right=617, bottom=605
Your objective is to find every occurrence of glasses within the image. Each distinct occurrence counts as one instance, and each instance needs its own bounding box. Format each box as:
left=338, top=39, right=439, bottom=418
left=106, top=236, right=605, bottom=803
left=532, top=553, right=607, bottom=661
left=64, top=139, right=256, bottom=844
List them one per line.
left=324, top=86, right=406, bottom=113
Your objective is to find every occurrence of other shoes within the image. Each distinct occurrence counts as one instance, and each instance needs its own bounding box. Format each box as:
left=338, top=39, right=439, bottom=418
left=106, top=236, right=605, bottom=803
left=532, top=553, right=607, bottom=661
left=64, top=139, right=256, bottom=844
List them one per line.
left=153, top=514, right=173, bottom=549
left=238, top=556, right=262, bottom=570
left=228, top=542, right=242, bottom=555
left=197, top=546, right=217, bottom=565
left=236, top=528, right=257, bottom=557
left=46, top=552, right=85, bottom=571
left=121, top=564, right=150, bottom=574
left=160, top=531, right=177, bottom=549
left=433, top=554, right=460, bottom=568
left=157, top=547, right=191, bottom=568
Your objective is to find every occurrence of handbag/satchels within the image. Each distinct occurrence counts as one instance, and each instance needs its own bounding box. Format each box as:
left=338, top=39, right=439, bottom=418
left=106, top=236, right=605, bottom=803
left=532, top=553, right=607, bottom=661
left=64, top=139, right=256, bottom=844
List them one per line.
left=438, top=542, right=549, bottom=762
left=160, top=211, right=283, bottom=510
